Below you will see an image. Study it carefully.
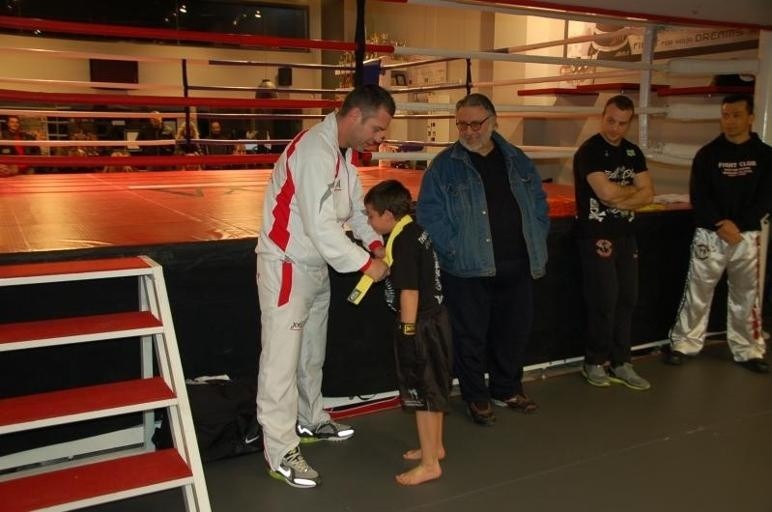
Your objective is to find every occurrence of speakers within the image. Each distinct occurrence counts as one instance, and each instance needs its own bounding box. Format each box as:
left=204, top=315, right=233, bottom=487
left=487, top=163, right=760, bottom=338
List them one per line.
left=278, top=68, right=292, bottom=85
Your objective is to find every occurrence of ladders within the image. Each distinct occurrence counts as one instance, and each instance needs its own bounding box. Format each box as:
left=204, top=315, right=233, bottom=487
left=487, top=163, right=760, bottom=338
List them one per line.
left=0, top=254, right=212, bottom=512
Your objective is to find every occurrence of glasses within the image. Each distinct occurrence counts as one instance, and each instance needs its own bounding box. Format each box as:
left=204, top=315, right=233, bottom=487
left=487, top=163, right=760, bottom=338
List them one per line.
left=455, top=112, right=494, bottom=132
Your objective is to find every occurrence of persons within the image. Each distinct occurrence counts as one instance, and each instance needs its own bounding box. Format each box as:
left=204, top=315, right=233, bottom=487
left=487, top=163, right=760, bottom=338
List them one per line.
left=253, top=84, right=399, bottom=492
left=61, top=110, right=256, bottom=173
left=571, top=96, right=655, bottom=393
left=363, top=178, right=451, bottom=486
left=659, top=95, right=771, bottom=374
left=417, top=93, right=551, bottom=425
left=1, top=115, right=44, bottom=175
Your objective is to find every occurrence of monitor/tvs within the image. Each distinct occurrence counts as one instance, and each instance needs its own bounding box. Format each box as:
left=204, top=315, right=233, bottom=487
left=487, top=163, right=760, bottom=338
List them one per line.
left=89, top=59, right=138, bottom=90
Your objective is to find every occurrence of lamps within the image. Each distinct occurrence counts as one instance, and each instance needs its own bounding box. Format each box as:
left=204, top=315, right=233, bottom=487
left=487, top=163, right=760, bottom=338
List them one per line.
left=251, top=52, right=279, bottom=100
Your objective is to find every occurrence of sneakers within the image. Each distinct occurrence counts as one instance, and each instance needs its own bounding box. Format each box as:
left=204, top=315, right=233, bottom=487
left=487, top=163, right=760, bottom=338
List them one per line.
left=665, top=348, right=703, bottom=366
left=295, top=419, right=357, bottom=445
left=580, top=359, right=613, bottom=388
left=729, top=356, right=772, bottom=374
left=262, top=445, right=323, bottom=491
left=487, top=391, right=543, bottom=415
left=606, top=361, right=652, bottom=391
left=465, top=397, right=497, bottom=428
